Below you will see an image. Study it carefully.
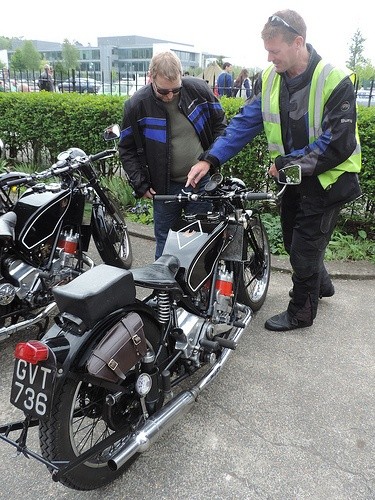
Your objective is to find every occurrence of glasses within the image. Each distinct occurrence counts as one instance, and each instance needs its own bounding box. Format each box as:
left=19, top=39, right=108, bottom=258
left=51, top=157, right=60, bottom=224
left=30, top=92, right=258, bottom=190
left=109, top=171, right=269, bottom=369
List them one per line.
left=154, top=82, right=183, bottom=96
left=268, top=15, right=305, bottom=39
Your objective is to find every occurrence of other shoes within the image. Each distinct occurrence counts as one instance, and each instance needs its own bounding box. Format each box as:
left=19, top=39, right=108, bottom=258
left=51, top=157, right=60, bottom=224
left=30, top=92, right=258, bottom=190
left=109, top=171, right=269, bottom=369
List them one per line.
left=289, top=287, right=335, bottom=297
left=264, top=310, right=314, bottom=332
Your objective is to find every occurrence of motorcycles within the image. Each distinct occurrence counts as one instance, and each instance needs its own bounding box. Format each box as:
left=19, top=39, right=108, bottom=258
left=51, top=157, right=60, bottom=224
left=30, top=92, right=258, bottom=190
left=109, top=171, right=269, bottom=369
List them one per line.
left=0, top=122, right=135, bottom=347
left=1, top=164, right=303, bottom=492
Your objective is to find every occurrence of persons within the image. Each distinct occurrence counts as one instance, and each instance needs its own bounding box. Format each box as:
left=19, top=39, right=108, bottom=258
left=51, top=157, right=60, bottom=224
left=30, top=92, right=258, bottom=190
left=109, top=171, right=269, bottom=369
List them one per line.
left=37, top=64, right=59, bottom=92
left=117, top=49, right=228, bottom=263
left=185, top=9, right=362, bottom=331
left=214, top=62, right=263, bottom=100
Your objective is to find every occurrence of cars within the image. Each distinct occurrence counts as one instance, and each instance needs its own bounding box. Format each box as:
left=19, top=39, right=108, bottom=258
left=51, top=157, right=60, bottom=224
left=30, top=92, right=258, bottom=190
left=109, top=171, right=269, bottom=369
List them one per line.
left=58, top=78, right=102, bottom=93
